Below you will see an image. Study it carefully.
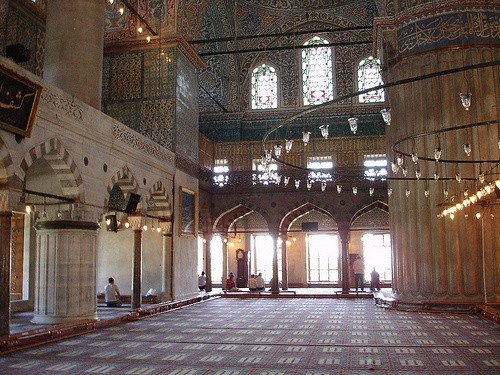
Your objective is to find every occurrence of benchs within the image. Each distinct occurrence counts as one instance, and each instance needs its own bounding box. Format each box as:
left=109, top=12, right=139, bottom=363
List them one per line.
left=223, top=291, right=296, bottom=294
left=335, top=291, right=367, bottom=296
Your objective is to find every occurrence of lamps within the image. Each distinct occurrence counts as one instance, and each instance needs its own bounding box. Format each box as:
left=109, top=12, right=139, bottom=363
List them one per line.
left=294, top=179, right=301, bottom=189
left=369, top=187, right=449, bottom=197
left=319, top=124, right=329, bottom=140
left=336, top=184, right=342, bottom=194
left=463, top=144, right=471, bottom=157
left=274, top=144, right=283, bottom=159
left=306, top=180, right=312, bottom=191
left=321, top=182, right=327, bottom=192
left=284, top=137, right=294, bottom=153
left=302, top=130, right=311, bottom=146
left=381, top=108, right=392, bottom=126
left=434, top=147, right=442, bottom=162
left=284, top=176, right=291, bottom=187
left=348, top=117, right=358, bottom=134
left=352, top=186, right=358, bottom=196
left=411, top=152, right=418, bottom=165
left=396, top=155, right=403, bottom=167
left=391, top=162, right=484, bottom=183
left=460, top=91, right=472, bottom=111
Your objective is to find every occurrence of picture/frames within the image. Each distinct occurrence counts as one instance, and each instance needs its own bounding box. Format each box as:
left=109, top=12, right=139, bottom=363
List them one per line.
left=0, top=64, right=42, bottom=137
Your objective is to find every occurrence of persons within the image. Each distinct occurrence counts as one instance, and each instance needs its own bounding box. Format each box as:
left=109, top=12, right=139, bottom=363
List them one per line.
left=247, top=274, right=258, bottom=292
left=353, top=255, right=365, bottom=291
left=198, top=272, right=207, bottom=291
left=104, top=277, right=122, bottom=306
left=370, top=267, right=379, bottom=290
left=255, top=273, right=265, bottom=290
left=269, top=277, right=279, bottom=291
left=227, top=274, right=238, bottom=291
